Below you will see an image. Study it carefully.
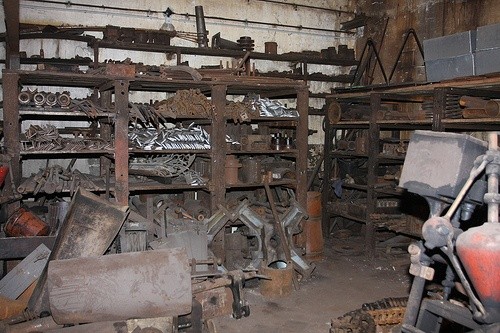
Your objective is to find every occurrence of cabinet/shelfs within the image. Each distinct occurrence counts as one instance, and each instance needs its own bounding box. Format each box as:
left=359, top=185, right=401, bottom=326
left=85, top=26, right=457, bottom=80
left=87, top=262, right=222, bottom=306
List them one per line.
left=1, top=69, right=309, bottom=259
left=321, top=73, right=499, bottom=268
